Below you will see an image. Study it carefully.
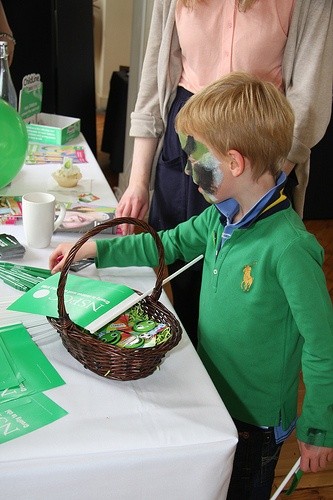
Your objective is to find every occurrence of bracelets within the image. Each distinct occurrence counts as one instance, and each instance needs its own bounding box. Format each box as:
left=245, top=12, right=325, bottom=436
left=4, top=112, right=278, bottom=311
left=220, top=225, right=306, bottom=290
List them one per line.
left=0, top=32, right=16, bottom=44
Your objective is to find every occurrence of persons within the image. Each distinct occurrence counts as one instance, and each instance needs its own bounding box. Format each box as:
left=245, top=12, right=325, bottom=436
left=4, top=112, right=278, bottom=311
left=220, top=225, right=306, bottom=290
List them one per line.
left=114, top=0, right=333, bottom=237
left=47, top=73, right=333, bottom=500
left=0, top=0, right=99, bottom=165
left=54, top=208, right=115, bottom=231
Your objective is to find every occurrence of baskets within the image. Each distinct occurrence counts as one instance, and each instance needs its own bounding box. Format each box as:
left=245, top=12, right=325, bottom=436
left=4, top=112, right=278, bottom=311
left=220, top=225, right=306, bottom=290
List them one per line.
left=44, top=216, right=182, bottom=382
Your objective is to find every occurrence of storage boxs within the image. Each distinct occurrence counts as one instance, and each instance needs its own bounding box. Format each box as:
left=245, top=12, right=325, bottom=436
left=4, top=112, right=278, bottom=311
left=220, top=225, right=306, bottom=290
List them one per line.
left=24, top=113, right=82, bottom=145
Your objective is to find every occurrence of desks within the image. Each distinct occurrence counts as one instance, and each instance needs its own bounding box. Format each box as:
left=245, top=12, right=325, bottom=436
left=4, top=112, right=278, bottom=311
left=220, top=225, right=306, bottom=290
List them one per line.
left=0, top=131, right=239, bottom=500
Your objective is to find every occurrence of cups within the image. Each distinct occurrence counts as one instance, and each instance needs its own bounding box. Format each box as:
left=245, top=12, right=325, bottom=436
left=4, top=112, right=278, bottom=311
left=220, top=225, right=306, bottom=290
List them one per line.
left=52, top=170, right=84, bottom=188
left=21, top=193, right=63, bottom=250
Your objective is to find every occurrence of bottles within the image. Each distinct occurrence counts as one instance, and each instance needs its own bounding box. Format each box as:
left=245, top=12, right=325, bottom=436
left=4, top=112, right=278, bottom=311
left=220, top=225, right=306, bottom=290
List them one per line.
left=0, top=42, right=18, bottom=113
